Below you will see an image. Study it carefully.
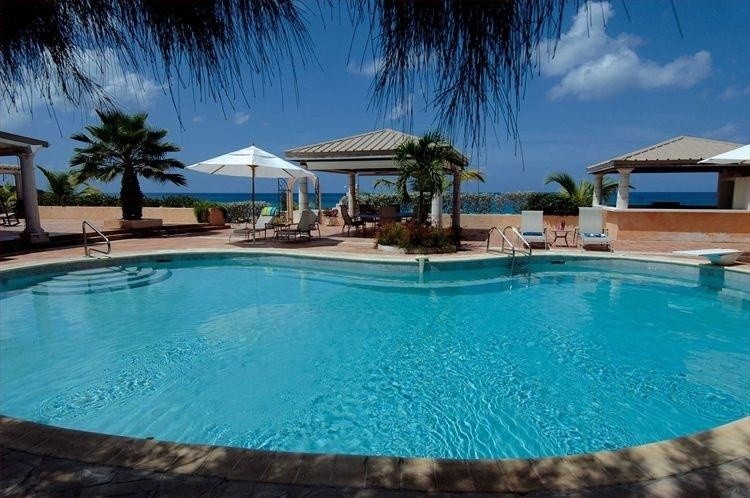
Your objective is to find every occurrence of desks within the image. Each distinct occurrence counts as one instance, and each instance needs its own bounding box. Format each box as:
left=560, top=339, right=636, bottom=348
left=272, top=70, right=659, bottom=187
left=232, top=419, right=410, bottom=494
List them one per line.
left=550, top=230, right=572, bottom=248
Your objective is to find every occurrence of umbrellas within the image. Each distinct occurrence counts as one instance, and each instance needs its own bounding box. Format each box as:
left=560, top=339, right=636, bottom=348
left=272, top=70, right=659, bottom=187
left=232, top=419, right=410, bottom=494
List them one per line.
left=184, top=142, right=317, bottom=243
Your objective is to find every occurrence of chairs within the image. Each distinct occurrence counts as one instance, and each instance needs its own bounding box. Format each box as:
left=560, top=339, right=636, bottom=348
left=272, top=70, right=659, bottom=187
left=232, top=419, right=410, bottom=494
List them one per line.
left=339, top=203, right=400, bottom=236
left=573, top=207, right=613, bottom=254
left=517, top=210, right=548, bottom=251
left=228, top=207, right=321, bottom=242
